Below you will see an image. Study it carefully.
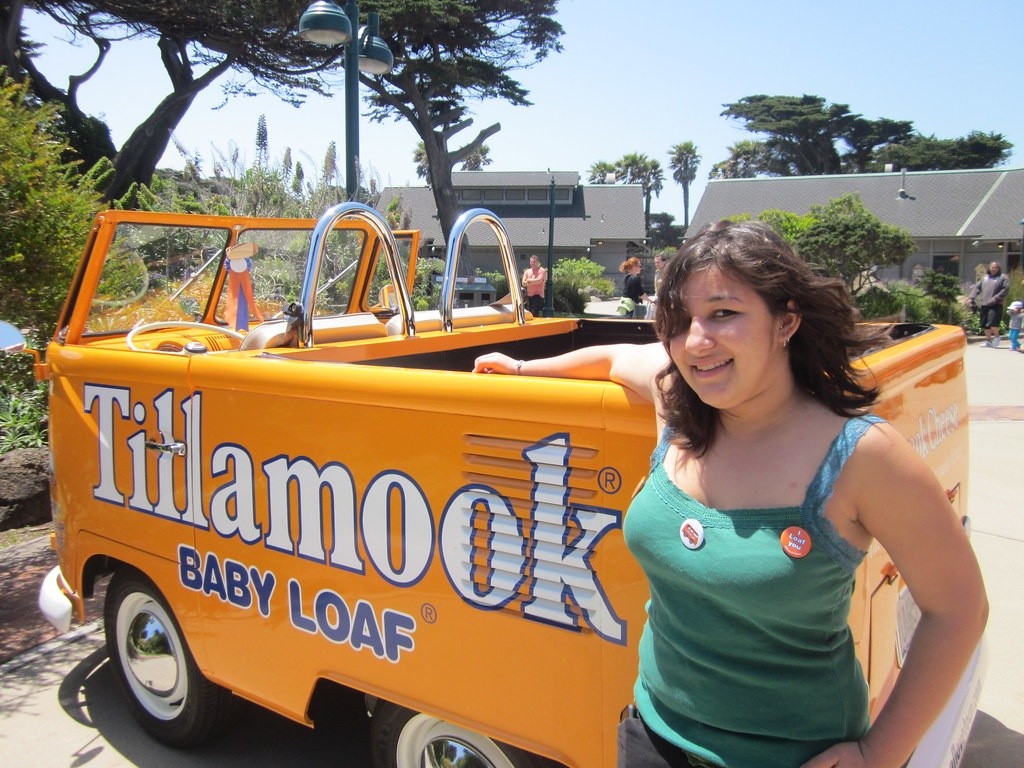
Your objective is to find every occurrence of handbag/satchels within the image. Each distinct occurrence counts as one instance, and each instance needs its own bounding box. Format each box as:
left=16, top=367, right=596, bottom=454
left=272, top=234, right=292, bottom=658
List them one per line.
left=632, top=304, right=647, bottom=319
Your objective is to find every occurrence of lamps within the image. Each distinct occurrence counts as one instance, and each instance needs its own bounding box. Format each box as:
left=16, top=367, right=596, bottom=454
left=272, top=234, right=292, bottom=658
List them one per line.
left=597, top=241, right=603, bottom=244
left=998, top=242, right=1004, bottom=249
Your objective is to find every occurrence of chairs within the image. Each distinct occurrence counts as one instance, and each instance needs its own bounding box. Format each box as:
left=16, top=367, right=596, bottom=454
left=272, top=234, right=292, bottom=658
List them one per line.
left=238, top=313, right=388, bottom=349
left=385, top=304, right=533, bottom=335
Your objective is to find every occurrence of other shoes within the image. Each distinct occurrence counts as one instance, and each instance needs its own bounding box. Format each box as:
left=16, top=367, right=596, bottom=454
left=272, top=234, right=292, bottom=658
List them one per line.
left=991, top=337, right=1000, bottom=347
left=1010, top=343, right=1021, bottom=351
left=980, top=341, right=992, bottom=347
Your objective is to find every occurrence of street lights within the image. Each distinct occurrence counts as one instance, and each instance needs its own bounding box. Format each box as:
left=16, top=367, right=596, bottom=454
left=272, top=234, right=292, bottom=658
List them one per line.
left=298, top=0, right=395, bottom=289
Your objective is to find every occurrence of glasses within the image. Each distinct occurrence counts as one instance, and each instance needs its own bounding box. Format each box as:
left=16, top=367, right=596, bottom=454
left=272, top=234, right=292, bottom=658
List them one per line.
left=638, top=265, right=642, bottom=268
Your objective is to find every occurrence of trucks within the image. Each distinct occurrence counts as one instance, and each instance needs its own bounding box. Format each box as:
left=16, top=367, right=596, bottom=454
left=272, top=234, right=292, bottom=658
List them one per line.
left=0, top=200, right=996, bottom=768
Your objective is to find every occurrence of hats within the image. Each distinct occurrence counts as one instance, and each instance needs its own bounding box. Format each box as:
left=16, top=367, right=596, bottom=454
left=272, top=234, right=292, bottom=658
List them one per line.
left=1007, top=301, right=1023, bottom=309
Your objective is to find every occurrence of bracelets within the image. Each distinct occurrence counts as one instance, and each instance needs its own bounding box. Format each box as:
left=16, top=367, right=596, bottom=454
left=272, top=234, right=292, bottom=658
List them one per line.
left=518, top=360, right=523, bottom=375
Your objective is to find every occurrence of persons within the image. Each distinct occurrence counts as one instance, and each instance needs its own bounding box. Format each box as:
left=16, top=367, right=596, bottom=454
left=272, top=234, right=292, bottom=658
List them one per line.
left=966, top=261, right=1010, bottom=347
left=937, top=264, right=944, bottom=273
left=654, top=254, right=666, bottom=305
left=1006, top=300, right=1024, bottom=351
left=472, top=218, right=991, bottom=768
left=522, top=254, right=547, bottom=317
left=425, top=282, right=434, bottom=296
left=616, top=256, right=652, bottom=320
